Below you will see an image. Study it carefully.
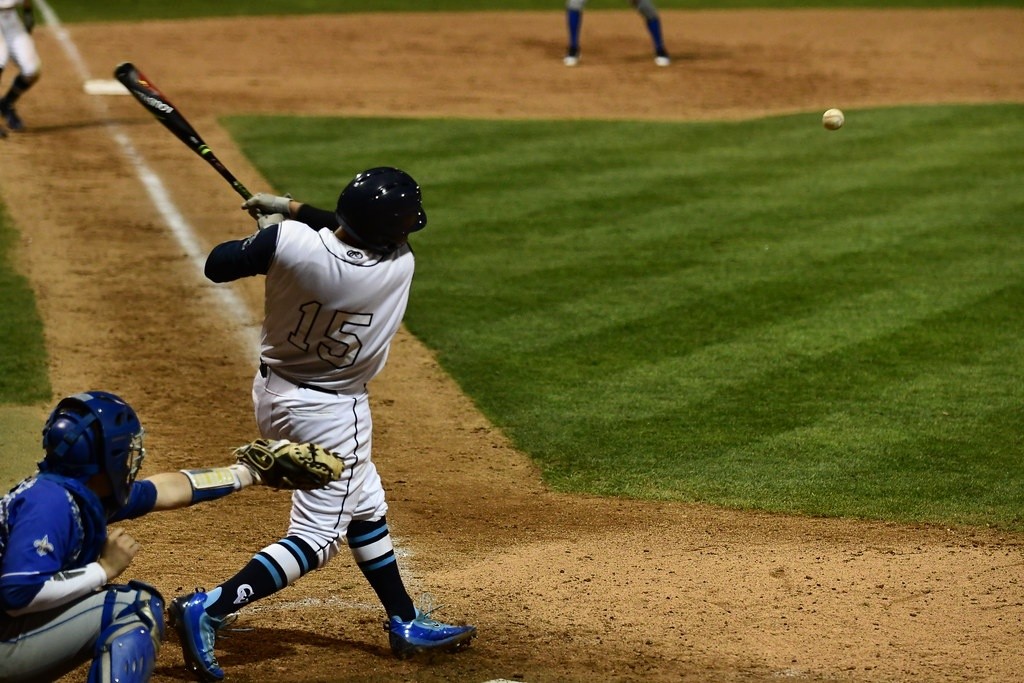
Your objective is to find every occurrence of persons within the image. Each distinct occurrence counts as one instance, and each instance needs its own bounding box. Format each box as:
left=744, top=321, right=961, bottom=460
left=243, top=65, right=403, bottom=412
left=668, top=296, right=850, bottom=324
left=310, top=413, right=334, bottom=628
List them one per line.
left=0, top=391, right=344, bottom=683
left=0, top=0, right=43, bottom=140
left=563, top=0, right=670, bottom=67
left=168, top=167, right=476, bottom=683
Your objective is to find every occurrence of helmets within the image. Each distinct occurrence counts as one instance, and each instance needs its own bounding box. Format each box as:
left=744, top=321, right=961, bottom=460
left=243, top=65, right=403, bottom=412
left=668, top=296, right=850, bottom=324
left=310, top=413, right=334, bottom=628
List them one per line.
left=45, top=391, right=144, bottom=508
left=335, top=167, right=427, bottom=254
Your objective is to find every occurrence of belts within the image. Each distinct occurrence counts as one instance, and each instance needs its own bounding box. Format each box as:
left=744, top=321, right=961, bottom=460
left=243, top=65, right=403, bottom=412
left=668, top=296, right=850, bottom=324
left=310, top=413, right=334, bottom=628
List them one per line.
left=257, top=359, right=337, bottom=395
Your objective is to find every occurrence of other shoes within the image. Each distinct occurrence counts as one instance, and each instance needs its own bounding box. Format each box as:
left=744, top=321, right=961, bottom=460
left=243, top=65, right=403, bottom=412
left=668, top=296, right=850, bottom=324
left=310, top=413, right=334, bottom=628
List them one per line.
left=565, top=47, right=580, bottom=67
left=0, top=96, right=23, bottom=129
left=655, top=54, right=669, bottom=67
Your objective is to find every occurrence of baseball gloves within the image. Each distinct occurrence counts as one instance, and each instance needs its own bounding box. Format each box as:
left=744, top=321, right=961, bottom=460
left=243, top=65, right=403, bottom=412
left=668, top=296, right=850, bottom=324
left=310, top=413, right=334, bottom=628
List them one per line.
left=232, top=438, right=345, bottom=493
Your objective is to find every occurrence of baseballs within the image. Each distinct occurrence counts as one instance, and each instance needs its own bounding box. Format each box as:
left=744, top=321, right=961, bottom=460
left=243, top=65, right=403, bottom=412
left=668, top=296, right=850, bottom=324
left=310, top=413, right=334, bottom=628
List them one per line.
left=822, top=107, right=844, bottom=130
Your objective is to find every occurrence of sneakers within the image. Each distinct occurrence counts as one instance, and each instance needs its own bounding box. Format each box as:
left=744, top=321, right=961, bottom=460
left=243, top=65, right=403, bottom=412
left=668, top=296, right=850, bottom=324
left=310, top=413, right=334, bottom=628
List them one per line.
left=383, top=594, right=476, bottom=659
left=168, top=586, right=253, bottom=679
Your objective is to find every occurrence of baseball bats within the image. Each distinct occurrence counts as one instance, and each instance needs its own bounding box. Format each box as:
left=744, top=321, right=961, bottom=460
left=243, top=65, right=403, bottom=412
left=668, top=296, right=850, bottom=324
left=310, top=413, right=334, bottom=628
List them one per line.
left=111, top=61, right=259, bottom=208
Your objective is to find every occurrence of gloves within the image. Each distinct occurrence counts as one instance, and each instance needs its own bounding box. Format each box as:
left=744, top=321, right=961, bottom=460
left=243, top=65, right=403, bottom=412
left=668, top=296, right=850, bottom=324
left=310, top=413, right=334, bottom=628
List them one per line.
left=241, top=193, right=294, bottom=220
left=256, top=192, right=290, bottom=229
